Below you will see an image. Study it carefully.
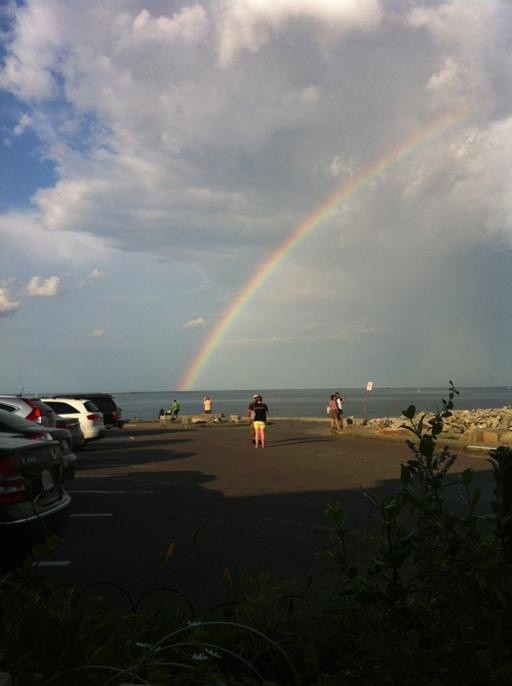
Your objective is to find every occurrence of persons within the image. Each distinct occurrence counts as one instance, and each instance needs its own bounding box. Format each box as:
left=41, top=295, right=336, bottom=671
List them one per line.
left=335, top=392, right=344, bottom=425
left=251, top=396, right=268, bottom=449
left=172, top=399, right=181, bottom=416
left=328, top=395, right=341, bottom=430
left=203, top=396, right=212, bottom=414
left=247, top=394, right=263, bottom=444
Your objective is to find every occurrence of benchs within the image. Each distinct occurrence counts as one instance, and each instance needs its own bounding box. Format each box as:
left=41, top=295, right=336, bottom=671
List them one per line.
left=229, top=414, right=272, bottom=424
left=160, top=413, right=223, bottom=425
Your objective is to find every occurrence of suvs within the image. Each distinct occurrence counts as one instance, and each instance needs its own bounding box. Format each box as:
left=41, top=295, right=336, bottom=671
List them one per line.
left=1, top=393, right=55, bottom=425
left=51, top=391, right=121, bottom=425
left=40, top=395, right=105, bottom=442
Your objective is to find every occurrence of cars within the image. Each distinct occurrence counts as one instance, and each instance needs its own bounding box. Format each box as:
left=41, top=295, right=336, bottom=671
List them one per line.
left=0, top=407, right=77, bottom=480
left=57, top=416, right=84, bottom=448
left=0, top=434, right=76, bottom=544
